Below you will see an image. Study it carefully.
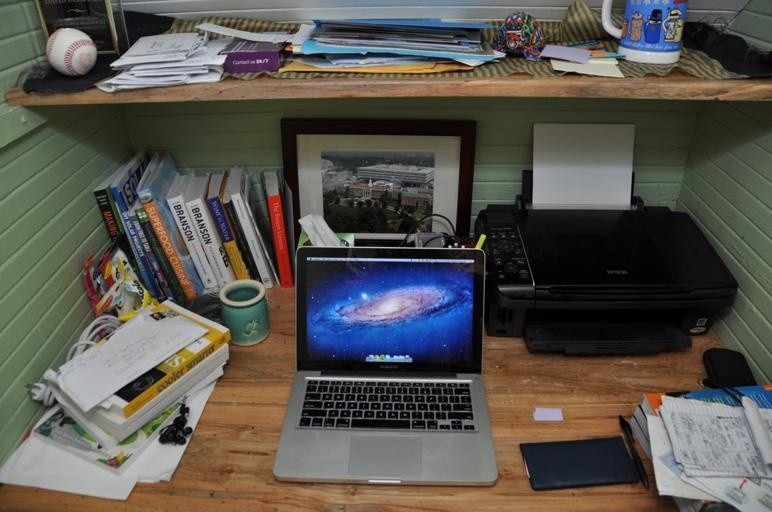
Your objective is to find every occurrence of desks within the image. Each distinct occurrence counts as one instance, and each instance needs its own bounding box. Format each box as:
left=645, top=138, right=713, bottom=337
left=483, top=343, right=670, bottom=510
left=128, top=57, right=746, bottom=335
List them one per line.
left=0, top=285, right=772, bottom=512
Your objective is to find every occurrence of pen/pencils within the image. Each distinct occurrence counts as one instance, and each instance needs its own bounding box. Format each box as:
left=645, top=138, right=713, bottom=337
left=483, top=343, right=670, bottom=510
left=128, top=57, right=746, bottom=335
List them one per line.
left=618, top=414, right=650, bottom=489
left=448, top=234, right=487, bottom=249
left=567, top=40, right=599, bottom=47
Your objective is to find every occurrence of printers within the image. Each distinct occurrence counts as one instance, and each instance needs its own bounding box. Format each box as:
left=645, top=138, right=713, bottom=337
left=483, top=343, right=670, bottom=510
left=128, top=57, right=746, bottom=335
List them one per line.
left=475, top=170, right=738, bottom=357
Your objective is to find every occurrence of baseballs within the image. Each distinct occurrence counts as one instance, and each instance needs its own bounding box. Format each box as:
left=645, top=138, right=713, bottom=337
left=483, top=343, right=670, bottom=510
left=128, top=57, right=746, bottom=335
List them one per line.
left=46, top=28, right=98, bottom=76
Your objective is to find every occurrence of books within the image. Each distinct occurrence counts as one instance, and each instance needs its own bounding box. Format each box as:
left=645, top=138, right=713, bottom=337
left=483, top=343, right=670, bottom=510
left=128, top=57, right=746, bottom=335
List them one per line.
left=33, top=300, right=231, bottom=472
left=519, top=435, right=640, bottom=491
left=626, top=383, right=772, bottom=511
left=93, top=149, right=295, bottom=303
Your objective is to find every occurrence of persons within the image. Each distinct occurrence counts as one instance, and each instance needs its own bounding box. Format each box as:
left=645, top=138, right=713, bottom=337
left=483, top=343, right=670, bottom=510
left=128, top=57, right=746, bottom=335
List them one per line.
left=322, top=180, right=433, bottom=234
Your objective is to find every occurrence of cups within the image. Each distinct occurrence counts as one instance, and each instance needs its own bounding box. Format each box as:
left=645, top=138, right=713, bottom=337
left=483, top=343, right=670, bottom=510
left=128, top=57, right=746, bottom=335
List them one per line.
left=218, top=278, right=271, bottom=345
left=601, top=0, right=687, bottom=65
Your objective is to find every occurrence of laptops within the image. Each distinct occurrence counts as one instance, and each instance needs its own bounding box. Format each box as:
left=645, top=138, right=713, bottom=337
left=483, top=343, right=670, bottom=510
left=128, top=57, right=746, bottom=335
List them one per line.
left=272, top=246, right=499, bottom=487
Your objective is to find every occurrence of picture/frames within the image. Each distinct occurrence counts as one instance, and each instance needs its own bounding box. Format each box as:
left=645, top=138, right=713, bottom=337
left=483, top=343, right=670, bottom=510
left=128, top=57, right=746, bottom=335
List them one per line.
left=280, top=119, right=477, bottom=260
